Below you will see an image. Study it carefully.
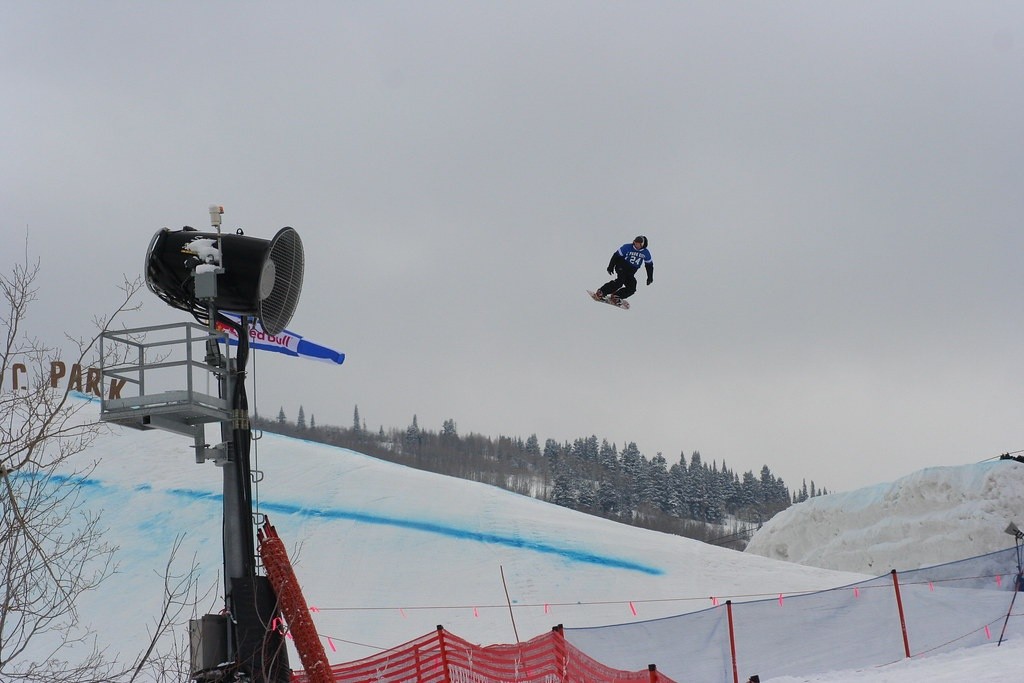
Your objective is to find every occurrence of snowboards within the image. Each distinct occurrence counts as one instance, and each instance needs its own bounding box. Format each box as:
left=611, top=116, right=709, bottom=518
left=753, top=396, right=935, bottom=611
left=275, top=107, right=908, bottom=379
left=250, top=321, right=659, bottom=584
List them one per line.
left=586, top=289, right=630, bottom=310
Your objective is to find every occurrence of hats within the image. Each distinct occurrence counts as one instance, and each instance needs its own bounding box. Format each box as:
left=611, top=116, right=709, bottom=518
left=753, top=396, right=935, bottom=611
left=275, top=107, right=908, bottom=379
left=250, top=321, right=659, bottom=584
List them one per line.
left=634, top=236, right=644, bottom=243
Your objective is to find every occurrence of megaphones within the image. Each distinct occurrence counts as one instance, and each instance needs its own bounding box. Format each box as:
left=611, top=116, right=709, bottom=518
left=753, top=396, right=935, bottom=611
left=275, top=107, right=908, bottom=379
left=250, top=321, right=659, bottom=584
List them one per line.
left=149, top=227, right=305, bottom=335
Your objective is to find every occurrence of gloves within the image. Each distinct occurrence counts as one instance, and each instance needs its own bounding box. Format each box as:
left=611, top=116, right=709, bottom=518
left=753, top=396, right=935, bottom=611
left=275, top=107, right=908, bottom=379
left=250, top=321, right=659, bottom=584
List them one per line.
left=647, top=277, right=653, bottom=285
left=607, top=267, right=614, bottom=275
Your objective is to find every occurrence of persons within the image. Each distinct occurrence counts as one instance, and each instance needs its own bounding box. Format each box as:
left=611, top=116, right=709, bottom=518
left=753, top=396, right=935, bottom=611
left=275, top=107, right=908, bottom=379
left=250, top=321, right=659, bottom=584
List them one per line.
left=596, top=236, right=654, bottom=306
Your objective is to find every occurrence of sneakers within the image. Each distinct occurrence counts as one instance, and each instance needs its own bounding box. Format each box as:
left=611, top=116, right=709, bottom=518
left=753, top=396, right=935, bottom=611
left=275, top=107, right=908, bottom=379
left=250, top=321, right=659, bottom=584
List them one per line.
left=611, top=294, right=622, bottom=307
left=596, top=289, right=606, bottom=302
